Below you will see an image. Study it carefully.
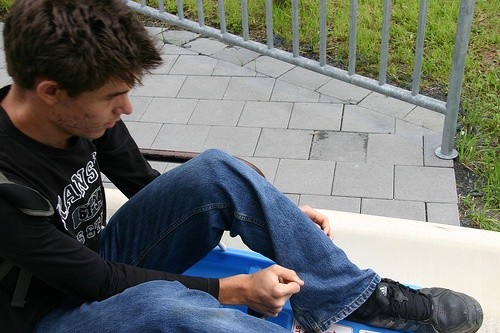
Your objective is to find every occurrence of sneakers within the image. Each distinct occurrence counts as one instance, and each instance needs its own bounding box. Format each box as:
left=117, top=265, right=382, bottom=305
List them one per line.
left=344, top=278, right=484, bottom=333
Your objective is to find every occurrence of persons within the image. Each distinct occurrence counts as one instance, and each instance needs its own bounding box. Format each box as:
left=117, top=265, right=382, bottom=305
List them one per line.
left=1, top=1, right=483, bottom=333
